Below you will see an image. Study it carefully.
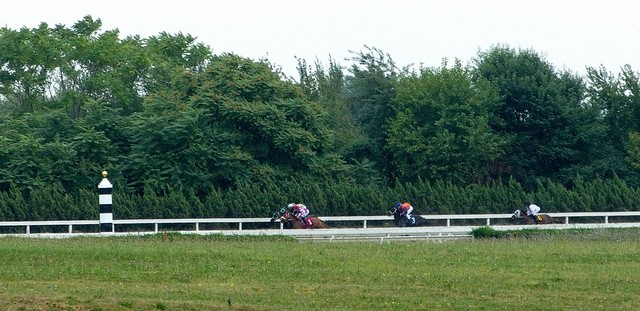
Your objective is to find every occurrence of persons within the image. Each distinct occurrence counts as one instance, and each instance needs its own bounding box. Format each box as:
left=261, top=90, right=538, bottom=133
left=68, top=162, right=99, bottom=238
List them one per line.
left=525, top=202, right=541, bottom=222
left=397, top=203, right=414, bottom=224
left=287, top=203, right=310, bottom=225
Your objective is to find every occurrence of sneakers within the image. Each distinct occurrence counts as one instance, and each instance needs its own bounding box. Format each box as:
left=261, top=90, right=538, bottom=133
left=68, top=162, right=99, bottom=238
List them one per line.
left=411, top=217, right=415, bottom=224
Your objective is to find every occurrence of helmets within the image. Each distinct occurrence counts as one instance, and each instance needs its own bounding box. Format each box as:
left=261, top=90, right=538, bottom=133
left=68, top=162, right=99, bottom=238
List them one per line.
left=524, top=202, right=530, bottom=208
left=287, top=203, right=294, bottom=208
left=396, top=202, right=402, bottom=208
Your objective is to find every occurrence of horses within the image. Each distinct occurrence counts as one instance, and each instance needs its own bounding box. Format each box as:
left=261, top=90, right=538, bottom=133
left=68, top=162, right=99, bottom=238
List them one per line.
left=271, top=210, right=293, bottom=226
left=511, top=209, right=562, bottom=225
left=280, top=209, right=329, bottom=229
left=386, top=206, right=431, bottom=227
